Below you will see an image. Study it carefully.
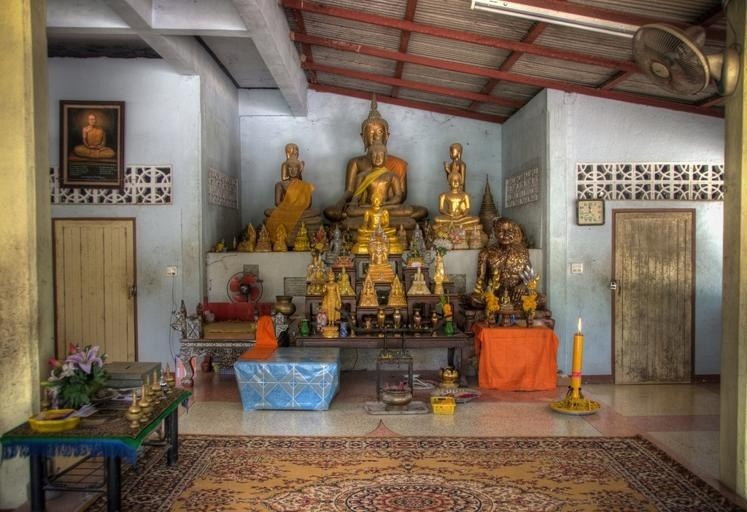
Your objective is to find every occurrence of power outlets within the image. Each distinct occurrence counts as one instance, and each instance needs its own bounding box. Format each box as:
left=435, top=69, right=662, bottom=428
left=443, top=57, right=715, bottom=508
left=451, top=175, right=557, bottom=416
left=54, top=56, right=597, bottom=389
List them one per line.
left=163, top=265, right=178, bottom=277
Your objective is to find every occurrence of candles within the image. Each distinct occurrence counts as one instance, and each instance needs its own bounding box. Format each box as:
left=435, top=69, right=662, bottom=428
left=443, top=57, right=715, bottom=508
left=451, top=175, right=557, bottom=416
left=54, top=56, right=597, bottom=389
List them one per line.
left=570, top=318, right=585, bottom=398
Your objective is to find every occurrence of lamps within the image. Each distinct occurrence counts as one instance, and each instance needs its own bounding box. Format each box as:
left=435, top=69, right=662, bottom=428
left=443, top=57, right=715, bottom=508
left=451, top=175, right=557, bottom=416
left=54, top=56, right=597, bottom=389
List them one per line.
left=470, top=0, right=641, bottom=39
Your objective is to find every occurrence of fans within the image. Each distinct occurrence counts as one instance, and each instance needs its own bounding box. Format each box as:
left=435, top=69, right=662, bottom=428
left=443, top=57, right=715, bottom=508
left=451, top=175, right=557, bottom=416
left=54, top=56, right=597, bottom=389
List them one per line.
left=630, top=24, right=737, bottom=98
left=226, top=271, right=262, bottom=324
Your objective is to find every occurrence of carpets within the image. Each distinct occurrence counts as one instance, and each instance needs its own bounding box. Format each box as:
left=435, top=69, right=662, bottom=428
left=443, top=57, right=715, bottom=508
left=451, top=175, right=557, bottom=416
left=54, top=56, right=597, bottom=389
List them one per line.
left=79, top=434, right=747, bottom=512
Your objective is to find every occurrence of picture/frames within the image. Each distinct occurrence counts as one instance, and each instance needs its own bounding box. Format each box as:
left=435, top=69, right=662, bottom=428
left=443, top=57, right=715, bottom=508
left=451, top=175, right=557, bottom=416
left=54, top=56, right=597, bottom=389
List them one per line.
left=58, top=100, right=125, bottom=190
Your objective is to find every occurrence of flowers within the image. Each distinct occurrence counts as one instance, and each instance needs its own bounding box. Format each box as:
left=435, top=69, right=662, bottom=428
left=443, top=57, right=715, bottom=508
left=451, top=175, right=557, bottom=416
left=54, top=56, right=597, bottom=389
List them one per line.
left=435, top=294, right=455, bottom=321
left=424, top=221, right=463, bottom=257
left=484, top=268, right=501, bottom=317
left=519, top=266, right=540, bottom=315
left=309, top=224, right=329, bottom=257
left=38, top=339, right=112, bottom=408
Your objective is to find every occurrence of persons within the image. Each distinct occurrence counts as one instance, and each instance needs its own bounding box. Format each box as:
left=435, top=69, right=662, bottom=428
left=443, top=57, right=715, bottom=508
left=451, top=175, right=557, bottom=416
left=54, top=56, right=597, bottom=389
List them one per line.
left=240, top=93, right=544, bottom=338
left=74, top=112, right=115, bottom=159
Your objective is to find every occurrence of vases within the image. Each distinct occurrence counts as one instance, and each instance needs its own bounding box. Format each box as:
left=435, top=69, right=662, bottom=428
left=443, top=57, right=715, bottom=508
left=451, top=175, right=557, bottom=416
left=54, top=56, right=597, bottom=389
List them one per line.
left=300, top=319, right=309, bottom=336
left=487, top=313, right=496, bottom=328
left=525, top=310, right=535, bottom=328
left=377, top=308, right=440, bottom=339
left=444, top=321, right=454, bottom=336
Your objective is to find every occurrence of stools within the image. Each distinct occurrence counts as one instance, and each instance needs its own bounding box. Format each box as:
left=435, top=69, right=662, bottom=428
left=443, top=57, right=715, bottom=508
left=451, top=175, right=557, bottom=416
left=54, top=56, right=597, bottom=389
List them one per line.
left=375, top=356, right=415, bottom=403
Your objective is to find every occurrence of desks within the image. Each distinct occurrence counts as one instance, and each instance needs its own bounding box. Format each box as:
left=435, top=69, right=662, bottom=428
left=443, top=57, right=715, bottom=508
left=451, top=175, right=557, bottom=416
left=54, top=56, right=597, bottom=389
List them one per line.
left=474, top=321, right=559, bottom=394
left=296, top=328, right=468, bottom=388
left=232, top=347, right=342, bottom=412
left=0, top=372, right=193, bottom=512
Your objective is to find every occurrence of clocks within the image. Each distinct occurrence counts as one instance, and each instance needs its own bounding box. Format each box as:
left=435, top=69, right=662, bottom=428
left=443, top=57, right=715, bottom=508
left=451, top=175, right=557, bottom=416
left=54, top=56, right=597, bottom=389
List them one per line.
left=576, top=199, right=605, bottom=226
